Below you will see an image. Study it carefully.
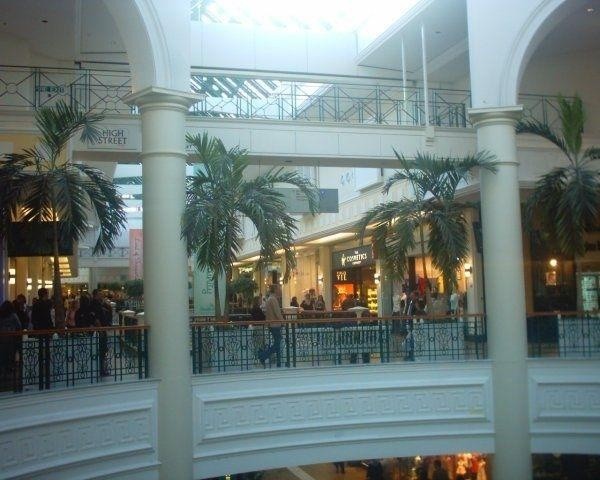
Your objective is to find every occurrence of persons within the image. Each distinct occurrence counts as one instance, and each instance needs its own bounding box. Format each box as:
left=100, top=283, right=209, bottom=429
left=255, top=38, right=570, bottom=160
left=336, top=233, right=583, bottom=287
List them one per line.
left=252, top=296, right=265, bottom=353
left=392, top=292, right=401, bottom=334
left=400, top=292, right=408, bottom=334
left=334, top=459, right=478, bottom=479
left=1, top=288, right=113, bottom=393
left=290, top=293, right=368, bottom=319
left=450, top=289, right=459, bottom=323
left=258, top=285, right=287, bottom=365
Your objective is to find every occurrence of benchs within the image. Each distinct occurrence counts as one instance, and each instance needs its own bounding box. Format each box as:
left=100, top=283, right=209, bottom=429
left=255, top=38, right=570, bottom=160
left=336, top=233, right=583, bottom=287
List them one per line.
left=557, top=318, right=600, bottom=357
left=191, top=322, right=464, bottom=367
